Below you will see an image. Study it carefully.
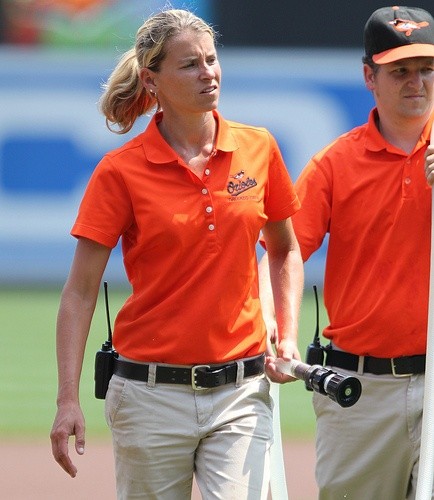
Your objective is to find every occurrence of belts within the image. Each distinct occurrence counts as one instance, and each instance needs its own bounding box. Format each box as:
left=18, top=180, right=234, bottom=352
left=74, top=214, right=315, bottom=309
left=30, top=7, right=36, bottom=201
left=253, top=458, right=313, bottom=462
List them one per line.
left=112, top=353, right=266, bottom=391
left=326, top=345, right=427, bottom=378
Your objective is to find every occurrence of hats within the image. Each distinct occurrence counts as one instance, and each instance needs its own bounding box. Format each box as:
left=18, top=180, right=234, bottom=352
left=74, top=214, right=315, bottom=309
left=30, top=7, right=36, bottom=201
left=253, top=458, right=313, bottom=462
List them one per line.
left=364, top=5, right=434, bottom=65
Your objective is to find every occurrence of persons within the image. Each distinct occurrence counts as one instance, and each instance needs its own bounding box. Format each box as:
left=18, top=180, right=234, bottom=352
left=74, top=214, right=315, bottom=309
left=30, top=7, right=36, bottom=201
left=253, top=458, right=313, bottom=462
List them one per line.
left=50, top=9, right=305, bottom=500
left=257, top=5, right=434, bottom=500
